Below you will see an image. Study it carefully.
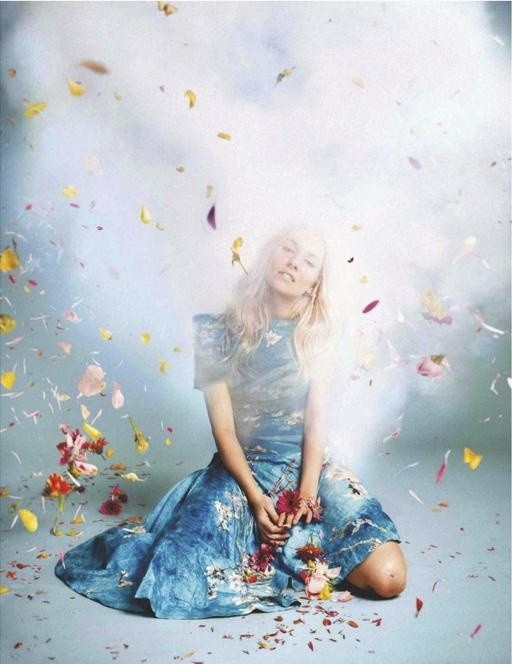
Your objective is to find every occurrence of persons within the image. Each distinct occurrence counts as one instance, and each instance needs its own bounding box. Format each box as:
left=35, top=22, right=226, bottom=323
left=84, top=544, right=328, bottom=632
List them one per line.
left=54, top=217, right=406, bottom=621
